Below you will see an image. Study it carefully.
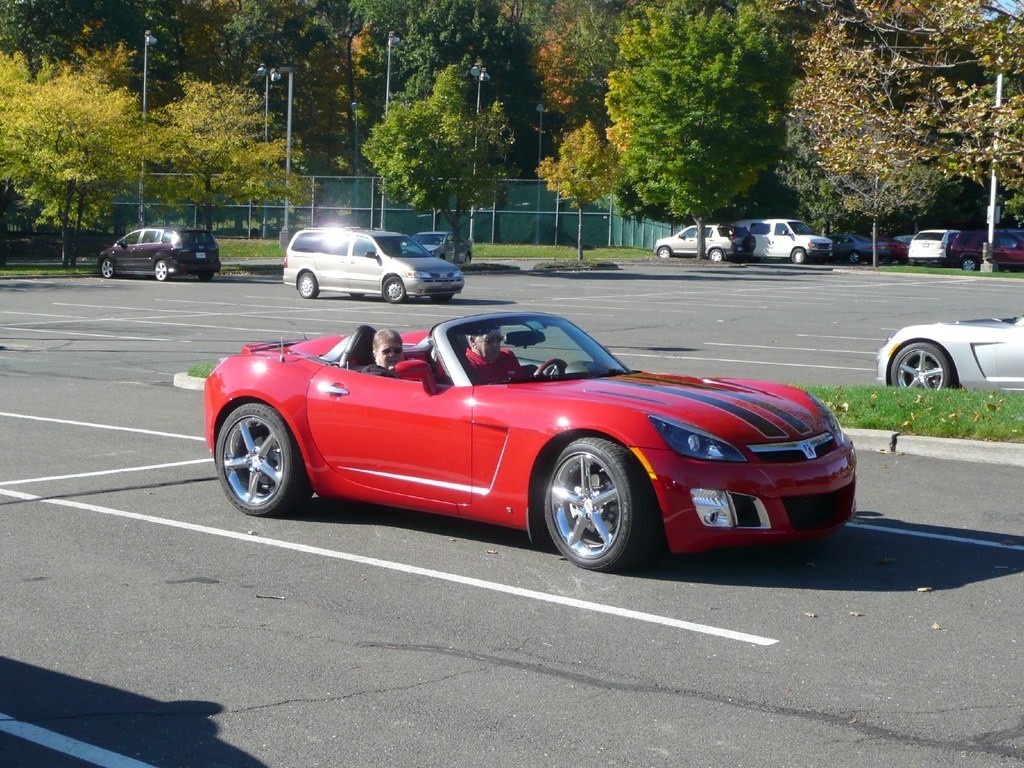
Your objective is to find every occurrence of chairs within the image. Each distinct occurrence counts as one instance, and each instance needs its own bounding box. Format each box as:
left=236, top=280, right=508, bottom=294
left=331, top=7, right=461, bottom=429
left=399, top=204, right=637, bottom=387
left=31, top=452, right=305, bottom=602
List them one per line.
left=426, top=334, right=471, bottom=382
left=339, top=324, right=378, bottom=372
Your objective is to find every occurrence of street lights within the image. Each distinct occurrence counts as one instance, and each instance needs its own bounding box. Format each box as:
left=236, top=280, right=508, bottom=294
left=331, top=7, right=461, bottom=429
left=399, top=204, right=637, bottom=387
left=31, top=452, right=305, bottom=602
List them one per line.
left=351, top=102, right=359, bottom=227
left=256, top=62, right=294, bottom=250
left=139, top=30, right=157, bottom=224
left=470, top=63, right=490, bottom=243
left=380, top=32, right=400, bottom=230
left=535, top=103, right=544, bottom=245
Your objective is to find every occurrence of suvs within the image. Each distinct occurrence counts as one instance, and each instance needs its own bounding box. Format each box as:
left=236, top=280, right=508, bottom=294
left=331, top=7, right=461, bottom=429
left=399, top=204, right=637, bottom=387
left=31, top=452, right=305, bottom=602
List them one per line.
left=947, top=229, right=1024, bottom=272
left=654, top=224, right=756, bottom=262
left=98, top=225, right=221, bottom=281
left=283, top=227, right=465, bottom=303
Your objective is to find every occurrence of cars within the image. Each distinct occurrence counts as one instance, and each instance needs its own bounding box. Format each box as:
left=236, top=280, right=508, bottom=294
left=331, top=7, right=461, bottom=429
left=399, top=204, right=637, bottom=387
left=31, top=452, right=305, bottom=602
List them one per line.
left=828, top=228, right=962, bottom=266
left=875, top=315, right=1024, bottom=393
left=412, top=231, right=472, bottom=264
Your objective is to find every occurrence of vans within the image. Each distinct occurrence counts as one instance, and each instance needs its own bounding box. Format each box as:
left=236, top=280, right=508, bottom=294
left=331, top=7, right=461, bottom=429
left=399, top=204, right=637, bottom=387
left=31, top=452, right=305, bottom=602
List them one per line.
left=731, top=219, right=833, bottom=264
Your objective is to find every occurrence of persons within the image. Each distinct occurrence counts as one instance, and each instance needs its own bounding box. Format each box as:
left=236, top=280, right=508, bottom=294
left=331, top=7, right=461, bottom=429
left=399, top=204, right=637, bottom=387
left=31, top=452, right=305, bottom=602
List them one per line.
left=366, top=328, right=410, bottom=379
left=463, top=329, right=528, bottom=383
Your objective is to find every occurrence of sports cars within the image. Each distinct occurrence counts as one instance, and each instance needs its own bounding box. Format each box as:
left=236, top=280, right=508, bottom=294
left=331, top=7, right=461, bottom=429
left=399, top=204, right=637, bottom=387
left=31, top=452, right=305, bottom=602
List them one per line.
left=203, top=311, right=857, bottom=573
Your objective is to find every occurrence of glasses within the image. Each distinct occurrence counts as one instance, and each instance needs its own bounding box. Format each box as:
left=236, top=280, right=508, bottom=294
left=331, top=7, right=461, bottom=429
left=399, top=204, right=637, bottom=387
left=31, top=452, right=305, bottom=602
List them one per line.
left=376, top=347, right=404, bottom=356
left=483, top=336, right=503, bottom=343
